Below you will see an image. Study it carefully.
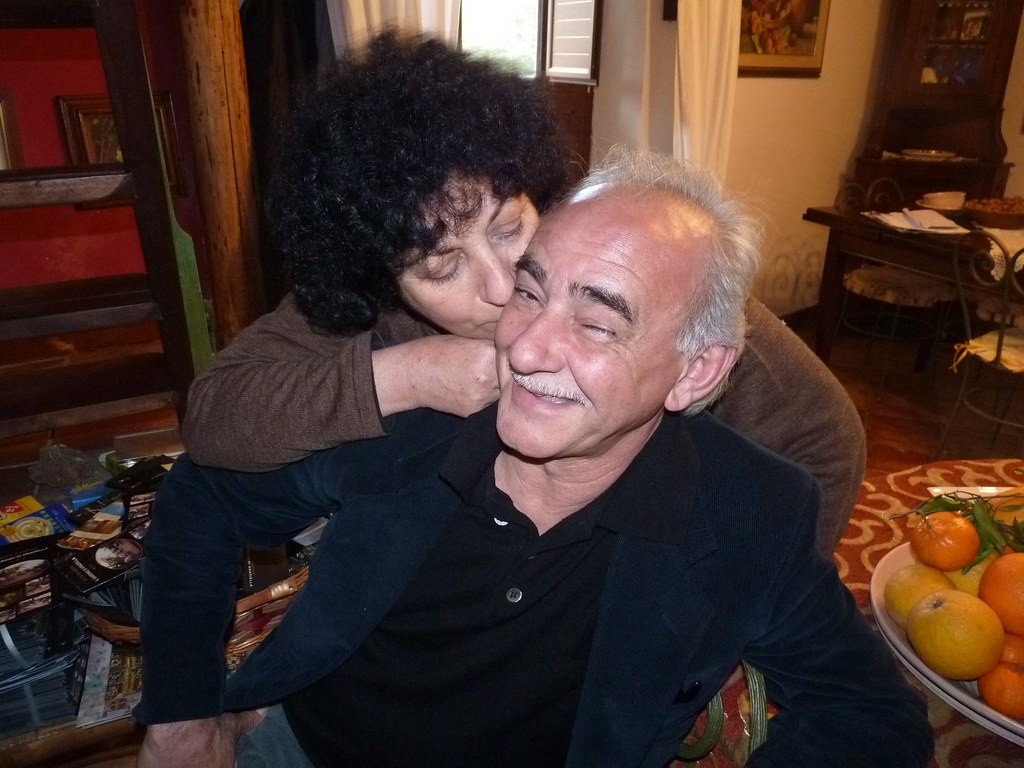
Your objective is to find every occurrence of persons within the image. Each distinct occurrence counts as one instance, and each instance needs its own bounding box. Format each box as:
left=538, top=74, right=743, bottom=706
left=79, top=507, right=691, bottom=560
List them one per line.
left=129, top=154, right=939, bottom=768
left=177, top=20, right=864, bottom=695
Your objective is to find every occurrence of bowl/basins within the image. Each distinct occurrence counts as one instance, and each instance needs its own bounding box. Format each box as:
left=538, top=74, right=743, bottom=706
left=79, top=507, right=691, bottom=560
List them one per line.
left=924, top=192, right=966, bottom=209
left=915, top=198, right=966, bottom=223
left=966, top=197, right=1024, bottom=229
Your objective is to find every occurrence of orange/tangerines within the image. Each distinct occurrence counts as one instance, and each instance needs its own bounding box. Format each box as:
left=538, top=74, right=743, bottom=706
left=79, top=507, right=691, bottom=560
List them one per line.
left=977, top=545, right=1024, bottom=720
left=910, top=511, right=982, bottom=571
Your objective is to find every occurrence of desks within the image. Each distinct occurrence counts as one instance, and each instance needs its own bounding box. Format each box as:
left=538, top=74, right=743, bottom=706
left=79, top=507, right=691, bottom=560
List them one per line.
left=804, top=206, right=1023, bottom=303
left=736, top=460, right=1024, bottom=768
left=0, top=408, right=189, bottom=768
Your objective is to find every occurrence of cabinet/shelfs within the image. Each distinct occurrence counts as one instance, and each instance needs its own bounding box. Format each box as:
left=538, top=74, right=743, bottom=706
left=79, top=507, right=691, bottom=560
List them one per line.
left=0, top=0, right=213, bottom=426
left=814, top=0, right=1024, bottom=361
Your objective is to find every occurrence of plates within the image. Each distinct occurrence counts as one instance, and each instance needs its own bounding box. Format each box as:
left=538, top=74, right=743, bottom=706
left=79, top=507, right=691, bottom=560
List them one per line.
left=869, top=542, right=1024, bottom=750
left=902, top=149, right=956, bottom=159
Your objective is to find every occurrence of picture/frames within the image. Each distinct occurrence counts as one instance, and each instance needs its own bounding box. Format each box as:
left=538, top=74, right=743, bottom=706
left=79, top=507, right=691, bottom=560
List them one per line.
left=53, top=91, right=184, bottom=212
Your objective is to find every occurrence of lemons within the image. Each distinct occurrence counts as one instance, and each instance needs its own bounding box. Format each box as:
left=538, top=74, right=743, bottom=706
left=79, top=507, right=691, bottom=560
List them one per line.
left=882, top=548, right=1007, bottom=680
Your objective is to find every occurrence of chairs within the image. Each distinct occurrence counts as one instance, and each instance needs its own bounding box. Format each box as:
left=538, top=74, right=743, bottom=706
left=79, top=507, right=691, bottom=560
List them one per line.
left=833, top=178, right=951, bottom=400
left=929, top=231, right=1024, bottom=460
